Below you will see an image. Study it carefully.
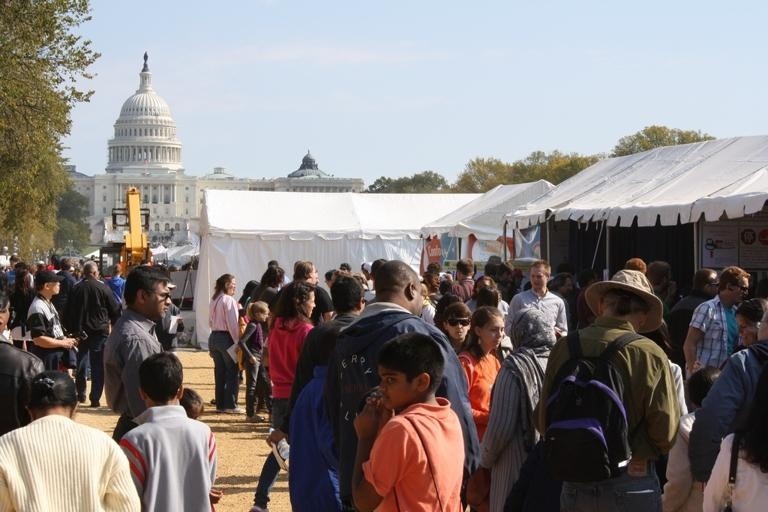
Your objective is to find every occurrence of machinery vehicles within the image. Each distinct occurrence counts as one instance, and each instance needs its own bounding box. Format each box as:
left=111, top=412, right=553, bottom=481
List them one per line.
left=86, top=178, right=156, bottom=280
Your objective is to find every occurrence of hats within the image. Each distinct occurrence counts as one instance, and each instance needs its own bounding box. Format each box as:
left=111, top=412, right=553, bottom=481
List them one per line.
left=584, top=268, right=665, bottom=334
left=167, top=282, right=177, bottom=289
left=0, top=258, right=45, bottom=268
left=33, top=270, right=67, bottom=284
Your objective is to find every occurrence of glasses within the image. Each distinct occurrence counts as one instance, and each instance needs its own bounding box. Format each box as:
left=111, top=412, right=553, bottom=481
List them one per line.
left=445, top=316, right=472, bottom=328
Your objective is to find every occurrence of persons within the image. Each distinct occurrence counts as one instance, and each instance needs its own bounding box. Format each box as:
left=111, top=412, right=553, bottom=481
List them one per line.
left=3, top=255, right=768, bottom=511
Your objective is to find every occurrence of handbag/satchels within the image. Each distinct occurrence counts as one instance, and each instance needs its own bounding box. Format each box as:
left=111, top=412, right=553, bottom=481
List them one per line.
left=61, top=347, right=79, bottom=370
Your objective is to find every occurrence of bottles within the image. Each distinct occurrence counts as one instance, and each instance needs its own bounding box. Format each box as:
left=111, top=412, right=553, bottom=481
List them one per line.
left=268, top=425, right=290, bottom=460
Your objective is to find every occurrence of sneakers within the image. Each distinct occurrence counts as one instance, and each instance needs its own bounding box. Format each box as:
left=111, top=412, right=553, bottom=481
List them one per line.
left=76, top=394, right=101, bottom=407
left=215, top=408, right=246, bottom=414
left=244, top=415, right=265, bottom=423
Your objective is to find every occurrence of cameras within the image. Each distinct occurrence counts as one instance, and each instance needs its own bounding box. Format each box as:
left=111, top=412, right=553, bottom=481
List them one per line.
left=65, top=332, right=88, bottom=369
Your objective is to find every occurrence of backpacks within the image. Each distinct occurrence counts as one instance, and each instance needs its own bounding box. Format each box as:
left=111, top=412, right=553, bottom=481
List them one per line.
left=542, top=331, right=643, bottom=483
